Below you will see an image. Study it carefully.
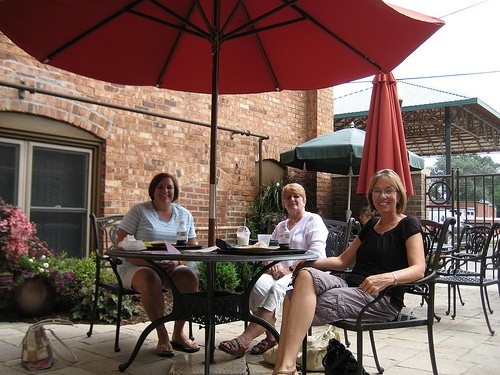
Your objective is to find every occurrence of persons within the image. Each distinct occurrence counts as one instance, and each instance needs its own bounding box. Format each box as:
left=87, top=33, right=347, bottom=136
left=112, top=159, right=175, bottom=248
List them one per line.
left=218, top=184, right=329, bottom=357
left=273, top=169, right=425, bottom=375
left=118, top=173, right=200, bottom=355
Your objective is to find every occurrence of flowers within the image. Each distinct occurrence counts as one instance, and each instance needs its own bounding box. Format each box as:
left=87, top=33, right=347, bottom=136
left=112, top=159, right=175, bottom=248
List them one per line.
left=0, top=196, right=78, bottom=292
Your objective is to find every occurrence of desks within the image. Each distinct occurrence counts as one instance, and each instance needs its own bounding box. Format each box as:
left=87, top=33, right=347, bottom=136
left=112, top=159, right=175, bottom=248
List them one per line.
left=104, top=246, right=320, bottom=375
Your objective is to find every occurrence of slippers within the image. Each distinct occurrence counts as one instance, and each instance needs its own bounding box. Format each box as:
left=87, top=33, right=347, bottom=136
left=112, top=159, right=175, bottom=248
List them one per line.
left=157, top=343, right=175, bottom=357
left=171, top=339, right=200, bottom=353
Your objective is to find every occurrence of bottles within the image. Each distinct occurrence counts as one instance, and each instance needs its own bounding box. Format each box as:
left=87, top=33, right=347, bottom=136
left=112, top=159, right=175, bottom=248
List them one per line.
left=278, top=221, right=290, bottom=249
left=177, top=221, right=188, bottom=246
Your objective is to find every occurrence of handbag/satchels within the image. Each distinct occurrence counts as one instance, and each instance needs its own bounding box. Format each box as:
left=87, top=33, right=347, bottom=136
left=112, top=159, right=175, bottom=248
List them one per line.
left=262, top=324, right=340, bottom=372
left=21, top=317, right=77, bottom=371
left=321, top=338, right=369, bottom=375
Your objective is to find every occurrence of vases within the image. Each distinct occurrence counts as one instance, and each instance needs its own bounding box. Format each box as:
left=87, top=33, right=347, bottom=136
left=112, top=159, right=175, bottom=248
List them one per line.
left=6, top=273, right=56, bottom=317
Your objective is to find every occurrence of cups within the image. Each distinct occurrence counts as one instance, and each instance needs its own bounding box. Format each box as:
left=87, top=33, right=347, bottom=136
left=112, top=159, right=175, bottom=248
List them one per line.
left=235, top=226, right=251, bottom=246
left=257, top=234, right=272, bottom=247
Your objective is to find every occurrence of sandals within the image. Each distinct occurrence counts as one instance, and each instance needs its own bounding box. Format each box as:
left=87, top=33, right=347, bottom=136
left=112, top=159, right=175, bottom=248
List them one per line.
left=251, top=338, right=277, bottom=354
left=219, top=338, right=249, bottom=357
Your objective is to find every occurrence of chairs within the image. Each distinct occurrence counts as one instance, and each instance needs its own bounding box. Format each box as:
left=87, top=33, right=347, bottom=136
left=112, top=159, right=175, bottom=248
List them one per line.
left=88, top=212, right=500, bottom=375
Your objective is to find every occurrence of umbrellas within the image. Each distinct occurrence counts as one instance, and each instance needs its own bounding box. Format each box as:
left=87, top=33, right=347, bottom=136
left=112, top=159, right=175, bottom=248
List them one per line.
left=280, top=122, right=425, bottom=224
left=0, top=0, right=446, bottom=374
left=355, top=72, right=414, bottom=199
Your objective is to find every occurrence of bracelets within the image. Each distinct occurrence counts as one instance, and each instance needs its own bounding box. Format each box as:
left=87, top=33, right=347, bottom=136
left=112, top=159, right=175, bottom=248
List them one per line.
left=392, top=271, right=397, bottom=284
